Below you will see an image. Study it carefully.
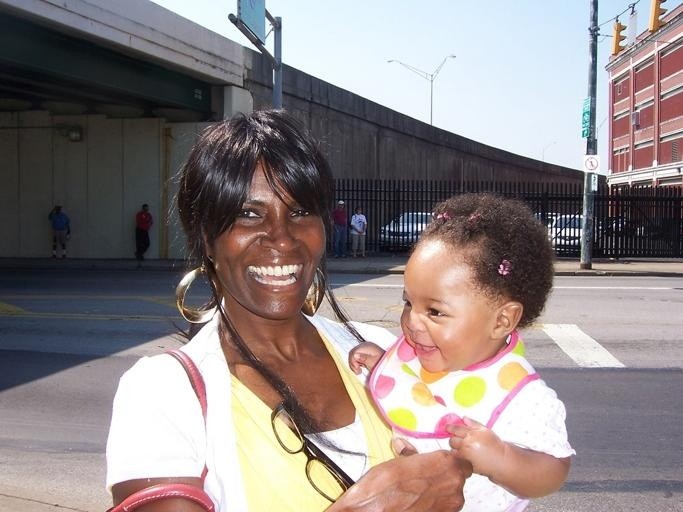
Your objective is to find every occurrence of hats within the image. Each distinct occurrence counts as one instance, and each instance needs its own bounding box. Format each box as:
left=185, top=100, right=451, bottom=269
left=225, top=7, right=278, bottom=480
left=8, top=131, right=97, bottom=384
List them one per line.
left=338, top=200, right=344, bottom=204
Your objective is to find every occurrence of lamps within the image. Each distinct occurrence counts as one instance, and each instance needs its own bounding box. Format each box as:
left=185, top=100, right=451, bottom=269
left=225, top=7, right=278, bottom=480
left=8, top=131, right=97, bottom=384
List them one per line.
left=67, top=125, right=84, bottom=141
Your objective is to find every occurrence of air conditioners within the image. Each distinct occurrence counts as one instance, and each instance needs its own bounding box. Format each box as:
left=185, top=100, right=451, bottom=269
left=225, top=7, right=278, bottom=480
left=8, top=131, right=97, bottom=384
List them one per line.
left=631, top=112, right=639, bottom=125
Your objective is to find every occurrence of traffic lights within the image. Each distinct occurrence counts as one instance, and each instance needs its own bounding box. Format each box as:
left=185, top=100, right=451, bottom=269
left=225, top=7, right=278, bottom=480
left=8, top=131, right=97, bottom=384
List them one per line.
left=647, top=0, right=666, bottom=32
left=611, top=21, right=627, bottom=56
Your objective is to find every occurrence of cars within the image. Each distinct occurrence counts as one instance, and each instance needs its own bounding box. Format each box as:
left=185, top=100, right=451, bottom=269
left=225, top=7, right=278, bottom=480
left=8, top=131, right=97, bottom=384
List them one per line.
left=378, top=211, right=436, bottom=249
left=545, top=213, right=601, bottom=256
left=607, top=218, right=627, bottom=235
left=533, top=211, right=561, bottom=228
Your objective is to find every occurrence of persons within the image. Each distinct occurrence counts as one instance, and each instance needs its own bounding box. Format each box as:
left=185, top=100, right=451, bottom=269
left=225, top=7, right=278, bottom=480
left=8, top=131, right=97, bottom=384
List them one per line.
left=47, top=203, right=71, bottom=259
left=348, top=206, right=367, bottom=258
left=94, top=111, right=477, bottom=511
left=331, top=200, right=347, bottom=258
left=132, top=203, right=152, bottom=261
left=348, top=192, right=576, bottom=511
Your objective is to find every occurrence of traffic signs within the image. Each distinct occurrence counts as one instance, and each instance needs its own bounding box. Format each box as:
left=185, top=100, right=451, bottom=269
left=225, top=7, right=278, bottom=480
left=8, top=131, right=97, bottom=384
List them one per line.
left=581, top=96, right=591, bottom=138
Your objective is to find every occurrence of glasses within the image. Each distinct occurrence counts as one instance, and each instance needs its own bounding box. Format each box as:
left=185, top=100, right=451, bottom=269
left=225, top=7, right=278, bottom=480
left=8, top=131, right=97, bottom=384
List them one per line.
left=271, top=404, right=356, bottom=503
